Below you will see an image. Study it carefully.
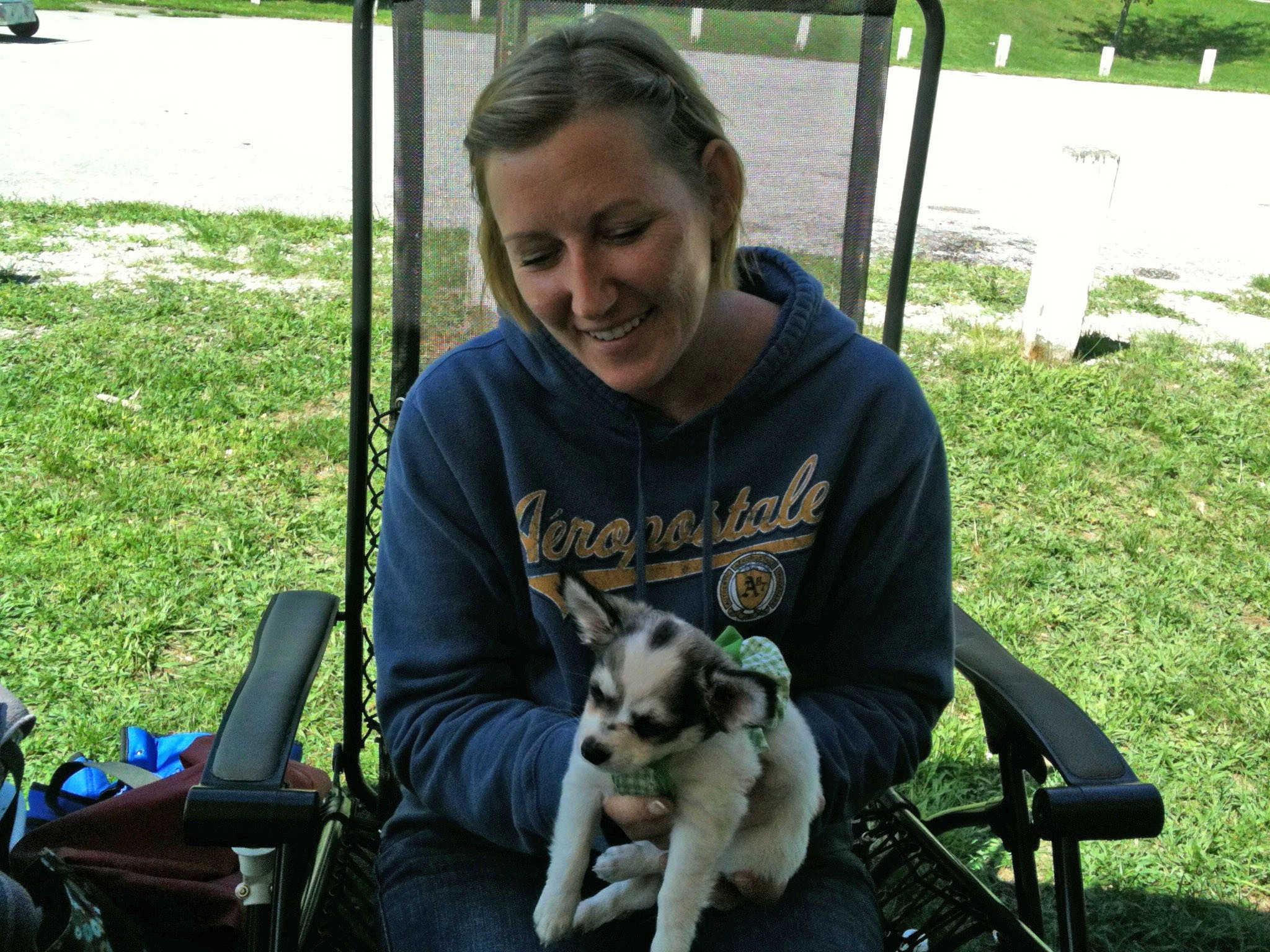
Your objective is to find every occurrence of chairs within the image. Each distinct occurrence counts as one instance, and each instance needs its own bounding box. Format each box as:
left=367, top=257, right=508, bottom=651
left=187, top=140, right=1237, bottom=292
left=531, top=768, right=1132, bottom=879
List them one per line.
left=184, top=0, right=1163, bottom=951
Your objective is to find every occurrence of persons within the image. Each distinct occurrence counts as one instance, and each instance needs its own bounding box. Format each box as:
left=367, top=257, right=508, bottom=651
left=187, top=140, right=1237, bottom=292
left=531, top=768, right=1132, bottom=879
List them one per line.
left=371, top=9, right=955, bottom=952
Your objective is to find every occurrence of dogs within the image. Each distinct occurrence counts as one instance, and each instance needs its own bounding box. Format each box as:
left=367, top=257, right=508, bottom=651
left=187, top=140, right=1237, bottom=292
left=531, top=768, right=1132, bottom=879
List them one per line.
left=529, top=567, right=823, bottom=952
